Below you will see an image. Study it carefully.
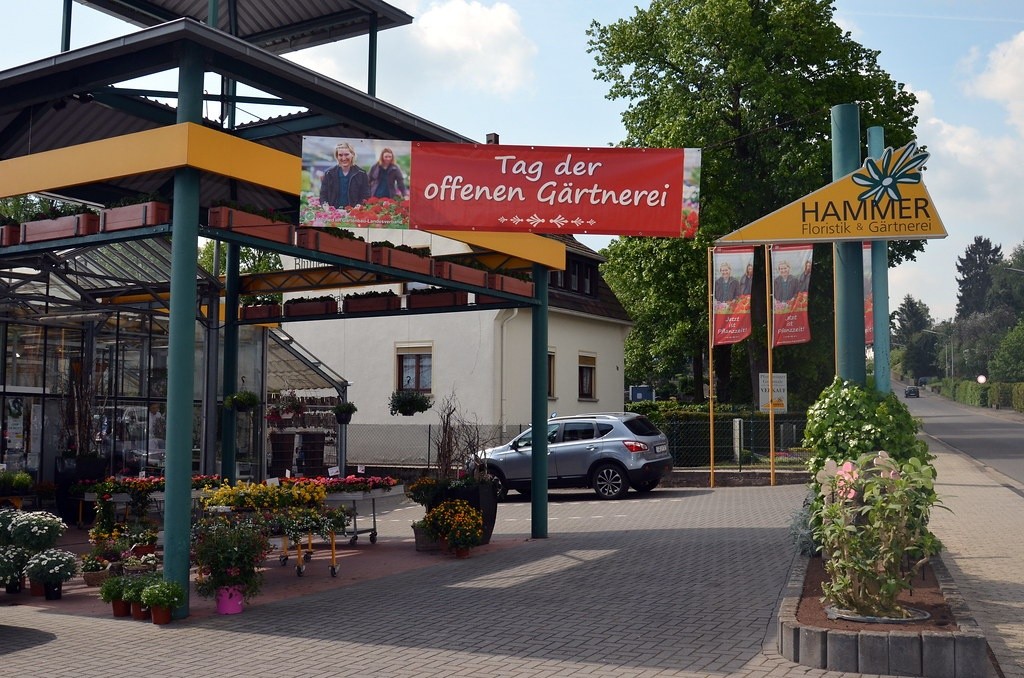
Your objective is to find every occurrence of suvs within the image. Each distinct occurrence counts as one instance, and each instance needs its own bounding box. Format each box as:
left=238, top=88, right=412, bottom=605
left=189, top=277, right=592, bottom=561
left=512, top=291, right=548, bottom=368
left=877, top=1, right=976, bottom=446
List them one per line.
left=464, top=410, right=674, bottom=503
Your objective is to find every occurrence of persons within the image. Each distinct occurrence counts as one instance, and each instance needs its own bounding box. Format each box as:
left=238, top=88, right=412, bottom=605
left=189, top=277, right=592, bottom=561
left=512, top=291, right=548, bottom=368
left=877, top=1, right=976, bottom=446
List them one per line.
left=146, top=402, right=161, bottom=449
left=714, top=260, right=812, bottom=303
left=319, top=143, right=371, bottom=209
left=368, top=148, right=407, bottom=198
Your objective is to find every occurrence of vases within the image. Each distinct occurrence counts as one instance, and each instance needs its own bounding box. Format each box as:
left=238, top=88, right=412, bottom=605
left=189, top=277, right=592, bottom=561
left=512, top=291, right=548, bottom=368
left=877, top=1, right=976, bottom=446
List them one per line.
left=44, top=580, right=62, bottom=600
left=5, top=578, right=21, bottom=594
left=152, top=604, right=172, bottom=625
left=132, top=543, right=155, bottom=558
left=131, top=602, right=152, bottom=620
left=107, top=562, right=123, bottom=578
left=448, top=482, right=498, bottom=547
left=414, top=526, right=441, bottom=552
left=31, top=580, right=45, bottom=597
left=19, top=212, right=101, bottom=243
left=125, top=567, right=156, bottom=579
left=440, top=535, right=470, bottom=559
left=11, top=575, right=25, bottom=589
left=83, top=570, right=110, bottom=588
left=112, top=597, right=131, bottom=617
left=266, top=392, right=338, bottom=430
left=213, top=584, right=245, bottom=614
left=824, top=601, right=932, bottom=624
left=210, top=207, right=295, bottom=245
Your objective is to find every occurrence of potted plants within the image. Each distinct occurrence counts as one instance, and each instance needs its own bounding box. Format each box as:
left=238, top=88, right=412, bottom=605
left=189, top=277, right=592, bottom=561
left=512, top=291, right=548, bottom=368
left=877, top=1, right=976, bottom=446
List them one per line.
left=98, top=192, right=169, bottom=233
left=332, top=401, right=359, bottom=425
left=386, top=389, right=436, bottom=418
left=296, top=224, right=533, bottom=299
left=0, top=193, right=30, bottom=248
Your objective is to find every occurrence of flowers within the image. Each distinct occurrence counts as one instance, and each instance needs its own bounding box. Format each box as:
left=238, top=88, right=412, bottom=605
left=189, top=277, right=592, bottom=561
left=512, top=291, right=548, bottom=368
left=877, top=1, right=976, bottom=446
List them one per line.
left=423, top=497, right=485, bottom=551
left=806, top=445, right=956, bottom=616
left=190, top=516, right=279, bottom=600
left=443, top=475, right=497, bottom=491
left=406, top=478, right=448, bottom=527
left=0, top=507, right=186, bottom=616
left=30, top=199, right=95, bottom=221
left=209, top=198, right=294, bottom=223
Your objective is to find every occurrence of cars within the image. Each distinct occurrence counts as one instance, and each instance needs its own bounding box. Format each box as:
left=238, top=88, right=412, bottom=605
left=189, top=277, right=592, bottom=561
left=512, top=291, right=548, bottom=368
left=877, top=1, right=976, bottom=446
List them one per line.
left=903, top=386, right=919, bottom=397
left=92, top=405, right=165, bottom=457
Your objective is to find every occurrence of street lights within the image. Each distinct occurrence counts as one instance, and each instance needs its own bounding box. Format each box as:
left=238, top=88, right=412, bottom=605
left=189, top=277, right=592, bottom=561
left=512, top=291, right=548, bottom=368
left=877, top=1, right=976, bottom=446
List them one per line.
left=923, top=328, right=954, bottom=382
